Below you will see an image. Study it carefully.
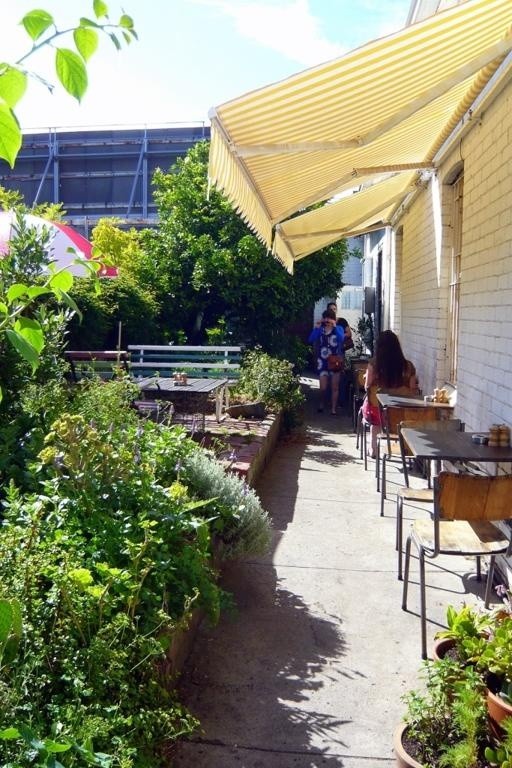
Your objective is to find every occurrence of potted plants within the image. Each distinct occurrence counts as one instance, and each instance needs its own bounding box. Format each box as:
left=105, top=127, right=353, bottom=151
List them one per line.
left=390, top=581, right=511, bottom=767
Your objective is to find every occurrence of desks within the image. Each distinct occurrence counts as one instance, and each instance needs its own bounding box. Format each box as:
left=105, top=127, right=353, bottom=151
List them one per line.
left=401, top=426, right=509, bottom=474
left=113, top=372, right=228, bottom=443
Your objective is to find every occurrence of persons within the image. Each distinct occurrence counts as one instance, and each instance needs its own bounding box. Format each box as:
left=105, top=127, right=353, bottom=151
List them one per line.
left=327, top=303, right=351, bottom=338
left=364, top=330, right=416, bottom=457
left=308, top=311, right=343, bottom=416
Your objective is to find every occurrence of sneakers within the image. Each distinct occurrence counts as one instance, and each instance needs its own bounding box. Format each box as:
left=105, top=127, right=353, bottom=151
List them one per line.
left=317, top=401, right=327, bottom=415
left=329, top=407, right=337, bottom=417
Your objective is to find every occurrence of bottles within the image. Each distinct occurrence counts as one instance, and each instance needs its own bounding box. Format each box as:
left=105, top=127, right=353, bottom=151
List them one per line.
left=441, top=387, right=448, bottom=402
left=433, top=388, right=440, bottom=402
left=488, top=425, right=499, bottom=447
left=173, top=371, right=187, bottom=384
left=498, top=424, right=510, bottom=447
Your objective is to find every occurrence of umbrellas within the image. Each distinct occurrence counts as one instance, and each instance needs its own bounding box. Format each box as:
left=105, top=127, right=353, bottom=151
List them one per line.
left=0, top=207, right=118, bottom=277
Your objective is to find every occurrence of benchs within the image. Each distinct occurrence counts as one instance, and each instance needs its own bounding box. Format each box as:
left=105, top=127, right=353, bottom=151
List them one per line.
left=125, top=345, right=246, bottom=419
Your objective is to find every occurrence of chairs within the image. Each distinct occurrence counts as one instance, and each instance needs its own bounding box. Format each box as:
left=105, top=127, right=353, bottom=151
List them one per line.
left=61, top=349, right=175, bottom=431
left=393, top=419, right=467, bottom=579
left=402, top=471, right=511, bottom=661
left=346, top=355, right=437, bottom=518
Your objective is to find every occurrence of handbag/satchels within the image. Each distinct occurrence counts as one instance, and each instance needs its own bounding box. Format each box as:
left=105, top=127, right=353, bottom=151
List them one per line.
left=326, top=353, right=345, bottom=372
